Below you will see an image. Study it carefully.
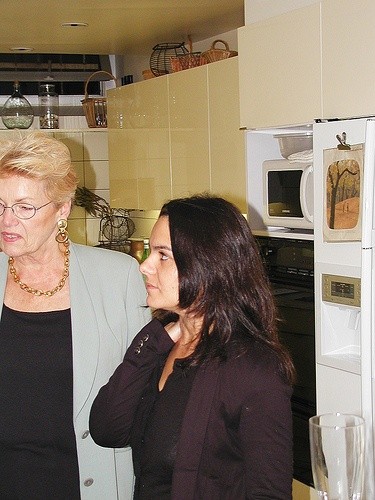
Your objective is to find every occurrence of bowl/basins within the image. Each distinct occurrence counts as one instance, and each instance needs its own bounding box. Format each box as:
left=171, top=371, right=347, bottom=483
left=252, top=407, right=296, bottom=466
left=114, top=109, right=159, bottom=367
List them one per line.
left=274, top=131, right=314, bottom=158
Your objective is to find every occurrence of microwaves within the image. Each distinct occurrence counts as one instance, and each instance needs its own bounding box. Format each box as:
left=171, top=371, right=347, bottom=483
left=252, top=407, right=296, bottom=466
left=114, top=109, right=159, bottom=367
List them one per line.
left=260, top=158, right=314, bottom=230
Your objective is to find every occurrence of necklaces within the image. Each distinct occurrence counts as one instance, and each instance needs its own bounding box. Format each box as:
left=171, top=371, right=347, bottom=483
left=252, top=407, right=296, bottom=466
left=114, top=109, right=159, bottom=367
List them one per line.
left=8, top=234, right=70, bottom=296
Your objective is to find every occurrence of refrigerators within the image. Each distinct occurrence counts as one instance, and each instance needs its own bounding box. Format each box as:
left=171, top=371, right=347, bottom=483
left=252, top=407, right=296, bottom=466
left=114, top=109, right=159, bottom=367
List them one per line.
left=311, top=116, right=375, bottom=499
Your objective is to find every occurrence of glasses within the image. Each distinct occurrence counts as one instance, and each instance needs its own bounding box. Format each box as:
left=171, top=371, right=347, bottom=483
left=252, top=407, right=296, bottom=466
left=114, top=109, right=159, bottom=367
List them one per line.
left=0, top=198, right=55, bottom=220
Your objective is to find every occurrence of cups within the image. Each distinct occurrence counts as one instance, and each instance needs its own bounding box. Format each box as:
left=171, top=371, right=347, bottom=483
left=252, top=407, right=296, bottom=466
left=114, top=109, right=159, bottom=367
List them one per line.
left=308, top=412, right=366, bottom=499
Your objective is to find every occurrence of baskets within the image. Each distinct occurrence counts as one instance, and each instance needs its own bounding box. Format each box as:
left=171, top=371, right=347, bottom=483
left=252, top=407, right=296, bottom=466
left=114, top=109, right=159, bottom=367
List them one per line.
left=201, top=39, right=238, bottom=63
left=80, top=70, right=119, bottom=128
left=170, top=35, right=203, bottom=73
left=143, top=69, right=169, bottom=80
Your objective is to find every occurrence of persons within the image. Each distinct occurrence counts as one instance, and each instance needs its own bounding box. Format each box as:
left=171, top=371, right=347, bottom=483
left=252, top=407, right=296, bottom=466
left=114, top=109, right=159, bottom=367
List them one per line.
left=88, top=190, right=297, bottom=500
left=0, top=128, right=153, bottom=500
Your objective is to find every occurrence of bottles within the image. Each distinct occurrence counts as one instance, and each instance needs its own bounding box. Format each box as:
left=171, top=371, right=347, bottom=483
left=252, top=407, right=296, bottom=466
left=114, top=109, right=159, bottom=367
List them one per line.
left=38, top=84, right=59, bottom=129
left=1, top=85, right=34, bottom=129
left=140, top=239, right=151, bottom=262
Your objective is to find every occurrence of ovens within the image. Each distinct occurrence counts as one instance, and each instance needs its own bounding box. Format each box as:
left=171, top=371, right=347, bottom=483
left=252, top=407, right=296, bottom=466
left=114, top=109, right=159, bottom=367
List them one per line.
left=252, top=235, right=315, bottom=488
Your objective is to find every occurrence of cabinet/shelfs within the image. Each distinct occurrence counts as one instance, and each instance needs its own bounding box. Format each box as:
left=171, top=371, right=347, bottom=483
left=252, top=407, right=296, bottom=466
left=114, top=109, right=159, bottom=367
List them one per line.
left=106, top=56, right=247, bottom=214
left=238, top=0, right=375, bottom=130
